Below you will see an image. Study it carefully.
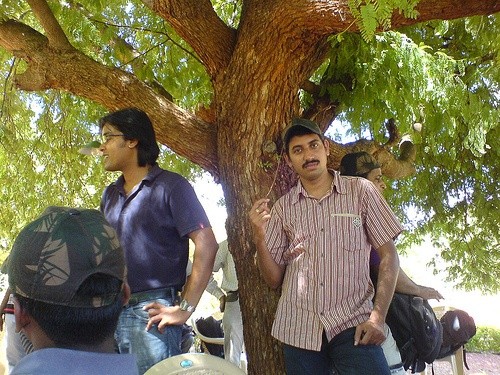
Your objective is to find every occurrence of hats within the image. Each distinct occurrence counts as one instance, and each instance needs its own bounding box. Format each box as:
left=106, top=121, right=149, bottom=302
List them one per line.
left=0, top=204, right=127, bottom=308
left=282, top=118, right=324, bottom=153
left=339, top=151, right=381, bottom=176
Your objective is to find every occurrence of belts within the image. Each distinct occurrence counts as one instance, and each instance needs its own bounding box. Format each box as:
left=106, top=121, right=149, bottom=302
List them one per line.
left=125, top=288, right=179, bottom=307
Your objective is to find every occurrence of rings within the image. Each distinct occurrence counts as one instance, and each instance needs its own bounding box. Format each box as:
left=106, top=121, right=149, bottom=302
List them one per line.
left=255, top=209, right=260, bottom=213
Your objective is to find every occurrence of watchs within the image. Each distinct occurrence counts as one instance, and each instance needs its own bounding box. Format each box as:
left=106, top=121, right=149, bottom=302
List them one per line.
left=180, top=299, right=195, bottom=312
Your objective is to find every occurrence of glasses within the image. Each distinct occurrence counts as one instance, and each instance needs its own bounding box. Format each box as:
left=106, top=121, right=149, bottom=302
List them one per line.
left=102, top=132, right=126, bottom=143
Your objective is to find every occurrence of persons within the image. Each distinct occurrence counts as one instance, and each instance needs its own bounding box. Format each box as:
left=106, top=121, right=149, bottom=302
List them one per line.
left=177, top=259, right=195, bottom=352
left=1, top=206, right=139, bottom=375
left=205, top=217, right=244, bottom=366
left=341, top=152, right=445, bottom=375
left=248, top=119, right=403, bottom=375
left=97, top=108, right=219, bottom=375
left=0, top=273, right=33, bottom=375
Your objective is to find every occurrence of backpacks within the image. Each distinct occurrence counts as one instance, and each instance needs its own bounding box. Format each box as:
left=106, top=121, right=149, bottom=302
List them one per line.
left=195, top=316, right=225, bottom=360
left=385, top=291, right=443, bottom=375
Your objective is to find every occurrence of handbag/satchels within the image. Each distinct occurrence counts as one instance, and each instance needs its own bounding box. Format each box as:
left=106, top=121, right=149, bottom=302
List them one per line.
left=435, top=310, right=476, bottom=370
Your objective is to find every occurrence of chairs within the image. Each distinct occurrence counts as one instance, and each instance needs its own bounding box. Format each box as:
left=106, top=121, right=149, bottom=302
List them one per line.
left=192, top=316, right=225, bottom=359
left=143, top=352, right=246, bottom=375
left=421, top=306, right=464, bottom=375
